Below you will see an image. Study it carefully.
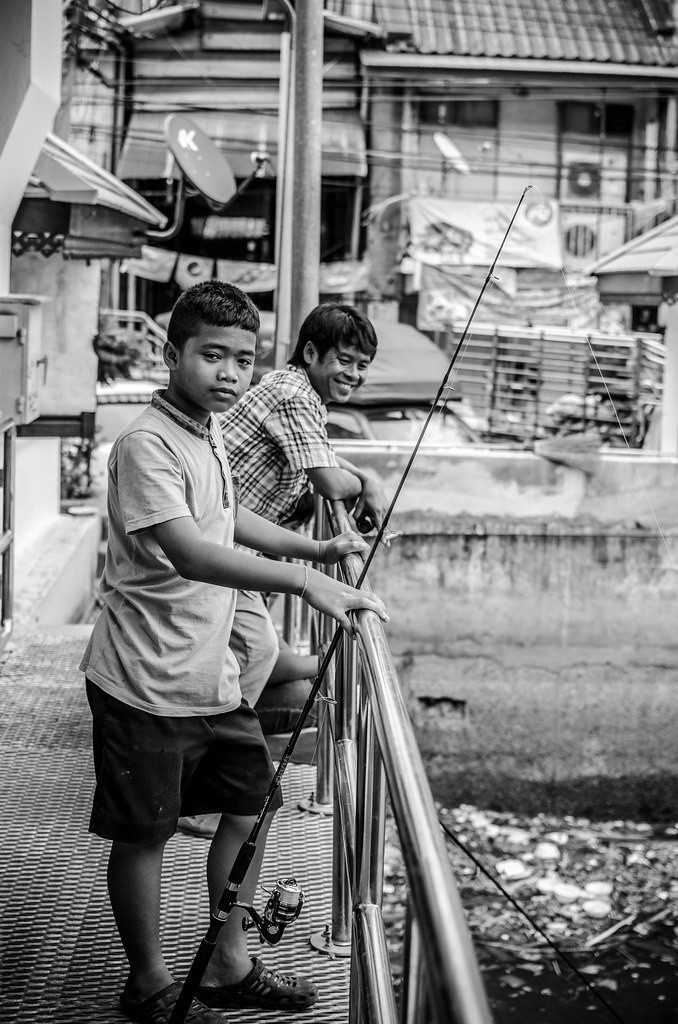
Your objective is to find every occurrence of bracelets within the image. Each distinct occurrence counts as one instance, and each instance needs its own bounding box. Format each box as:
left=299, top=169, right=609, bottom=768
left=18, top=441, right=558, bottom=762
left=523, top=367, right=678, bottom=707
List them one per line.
left=300, top=565, right=309, bottom=597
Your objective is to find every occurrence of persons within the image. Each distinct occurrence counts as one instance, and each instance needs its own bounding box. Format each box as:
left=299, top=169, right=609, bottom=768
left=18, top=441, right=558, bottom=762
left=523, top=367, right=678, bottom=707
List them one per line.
left=79, top=277, right=390, bottom=1024
left=178, top=302, right=388, bottom=840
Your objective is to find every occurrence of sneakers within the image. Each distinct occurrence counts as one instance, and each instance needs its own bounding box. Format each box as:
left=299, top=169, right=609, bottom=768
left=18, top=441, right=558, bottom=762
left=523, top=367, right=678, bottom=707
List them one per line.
left=177, top=812, right=221, bottom=837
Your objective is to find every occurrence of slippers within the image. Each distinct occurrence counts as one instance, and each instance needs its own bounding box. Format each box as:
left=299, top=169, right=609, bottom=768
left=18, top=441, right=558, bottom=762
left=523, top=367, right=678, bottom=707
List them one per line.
left=120, top=981, right=231, bottom=1024
left=196, top=957, right=319, bottom=1010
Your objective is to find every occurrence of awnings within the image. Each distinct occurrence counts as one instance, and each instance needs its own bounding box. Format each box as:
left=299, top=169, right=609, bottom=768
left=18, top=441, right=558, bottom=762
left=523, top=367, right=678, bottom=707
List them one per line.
left=117, top=113, right=369, bottom=180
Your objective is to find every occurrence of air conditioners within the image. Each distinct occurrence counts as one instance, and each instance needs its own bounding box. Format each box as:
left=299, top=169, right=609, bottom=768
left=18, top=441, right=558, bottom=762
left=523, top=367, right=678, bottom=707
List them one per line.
left=552, top=131, right=629, bottom=279
